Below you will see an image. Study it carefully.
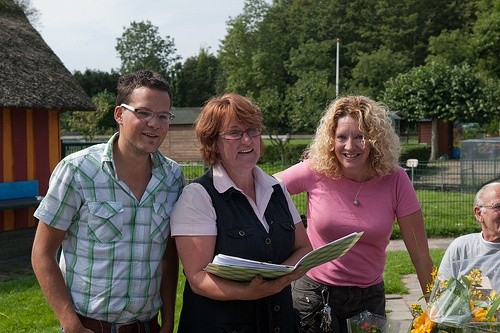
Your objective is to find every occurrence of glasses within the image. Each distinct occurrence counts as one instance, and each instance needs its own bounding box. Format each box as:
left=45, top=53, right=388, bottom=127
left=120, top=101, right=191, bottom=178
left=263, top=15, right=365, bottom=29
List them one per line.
left=224, top=126, right=262, bottom=140
left=120, top=103, right=176, bottom=123
left=478, top=203, right=500, bottom=211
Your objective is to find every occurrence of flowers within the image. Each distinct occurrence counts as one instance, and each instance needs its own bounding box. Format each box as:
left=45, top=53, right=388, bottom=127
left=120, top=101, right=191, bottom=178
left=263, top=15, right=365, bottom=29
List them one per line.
left=346, top=264, right=500, bottom=333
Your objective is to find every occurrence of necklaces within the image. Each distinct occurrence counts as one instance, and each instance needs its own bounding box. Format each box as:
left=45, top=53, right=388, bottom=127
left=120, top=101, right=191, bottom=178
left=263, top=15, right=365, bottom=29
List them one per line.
left=340, top=166, right=369, bottom=205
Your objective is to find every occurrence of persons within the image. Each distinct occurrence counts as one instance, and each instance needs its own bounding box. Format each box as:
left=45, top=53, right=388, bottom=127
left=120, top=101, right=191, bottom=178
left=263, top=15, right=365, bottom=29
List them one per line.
left=31, top=69, right=186, bottom=333
left=428, top=182, right=500, bottom=310
left=270, top=96, right=437, bottom=333
left=171, top=94, right=314, bottom=333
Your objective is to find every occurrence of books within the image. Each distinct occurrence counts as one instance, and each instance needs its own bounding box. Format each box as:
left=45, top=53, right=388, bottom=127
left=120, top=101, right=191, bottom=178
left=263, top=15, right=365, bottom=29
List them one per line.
left=203, top=232, right=365, bottom=280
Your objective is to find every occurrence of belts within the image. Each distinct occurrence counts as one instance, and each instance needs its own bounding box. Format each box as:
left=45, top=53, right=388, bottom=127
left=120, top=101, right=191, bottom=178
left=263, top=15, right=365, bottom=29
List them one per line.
left=74, top=312, right=161, bottom=333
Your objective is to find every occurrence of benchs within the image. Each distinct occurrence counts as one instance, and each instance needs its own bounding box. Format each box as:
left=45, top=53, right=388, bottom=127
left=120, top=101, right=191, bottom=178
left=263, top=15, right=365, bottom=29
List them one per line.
left=0, top=179, right=44, bottom=209
